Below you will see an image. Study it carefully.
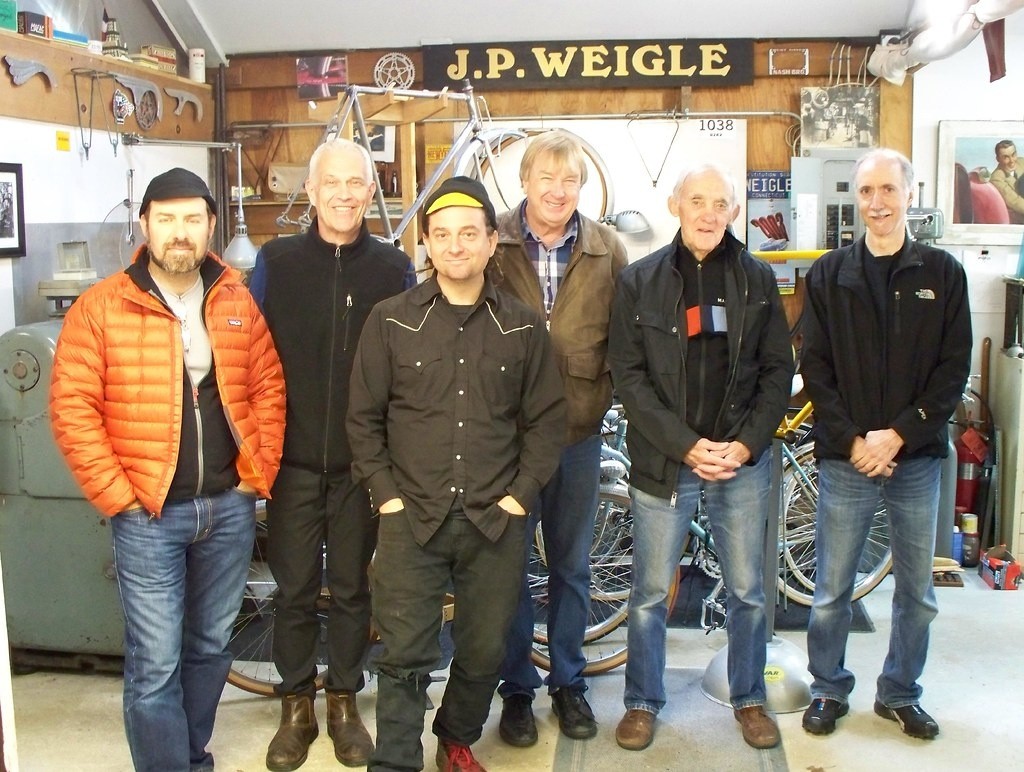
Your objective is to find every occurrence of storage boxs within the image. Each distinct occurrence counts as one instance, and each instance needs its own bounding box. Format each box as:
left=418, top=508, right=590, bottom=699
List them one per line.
left=129, top=53, right=158, bottom=70
left=17, top=10, right=53, bottom=40
left=140, top=43, right=178, bottom=75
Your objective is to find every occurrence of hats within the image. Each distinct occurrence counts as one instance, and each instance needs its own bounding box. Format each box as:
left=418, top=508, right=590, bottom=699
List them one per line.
left=421, top=175, right=497, bottom=233
left=139, top=167, right=216, bottom=218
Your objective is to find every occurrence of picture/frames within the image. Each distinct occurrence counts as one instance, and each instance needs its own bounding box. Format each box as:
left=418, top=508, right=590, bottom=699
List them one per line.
left=0, top=162, right=27, bottom=259
left=353, top=123, right=396, bottom=163
left=933, top=120, right=1024, bottom=247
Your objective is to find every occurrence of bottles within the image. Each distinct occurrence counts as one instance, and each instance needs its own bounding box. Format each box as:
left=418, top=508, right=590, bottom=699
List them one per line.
left=963, top=534, right=980, bottom=567
left=188, top=48, right=206, bottom=84
left=953, top=526, right=963, bottom=564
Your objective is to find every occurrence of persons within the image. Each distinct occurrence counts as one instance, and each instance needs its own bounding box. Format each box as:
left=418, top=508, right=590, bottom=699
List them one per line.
left=607, top=162, right=795, bottom=750
left=488, top=130, right=630, bottom=747
left=347, top=176, right=569, bottom=772
left=49, top=167, right=287, bottom=772
left=0, top=193, right=14, bottom=238
left=251, top=138, right=417, bottom=772
left=800, top=148, right=973, bottom=739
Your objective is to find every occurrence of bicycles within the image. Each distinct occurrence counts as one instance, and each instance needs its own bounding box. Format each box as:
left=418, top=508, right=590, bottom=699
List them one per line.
left=276, top=80, right=613, bottom=254
left=229, top=361, right=893, bottom=700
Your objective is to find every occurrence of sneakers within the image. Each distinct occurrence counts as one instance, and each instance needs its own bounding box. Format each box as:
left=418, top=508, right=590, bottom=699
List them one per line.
left=499, top=694, right=538, bottom=748
left=550, top=685, right=597, bottom=740
left=802, top=696, right=849, bottom=737
left=873, top=698, right=939, bottom=739
left=615, top=708, right=657, bottom=751
left=734, top=705, right=779, bottom=749
left=436, top=737, right=485, bottom=772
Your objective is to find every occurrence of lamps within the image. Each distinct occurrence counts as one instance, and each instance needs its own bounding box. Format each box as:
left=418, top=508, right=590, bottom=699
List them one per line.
left=605, top=209, right=651, bottom=234
left=122, top=133, right=258, bottom=270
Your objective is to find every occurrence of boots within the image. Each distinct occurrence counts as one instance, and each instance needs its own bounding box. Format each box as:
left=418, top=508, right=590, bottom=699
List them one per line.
left=266, top=693, right=319, bottom=772
left=326, top=691, right=376, bottom=767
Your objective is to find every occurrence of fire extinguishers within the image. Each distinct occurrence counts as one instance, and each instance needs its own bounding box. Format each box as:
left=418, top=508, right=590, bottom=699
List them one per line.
left=948, top=419, right=990, bottom=513
